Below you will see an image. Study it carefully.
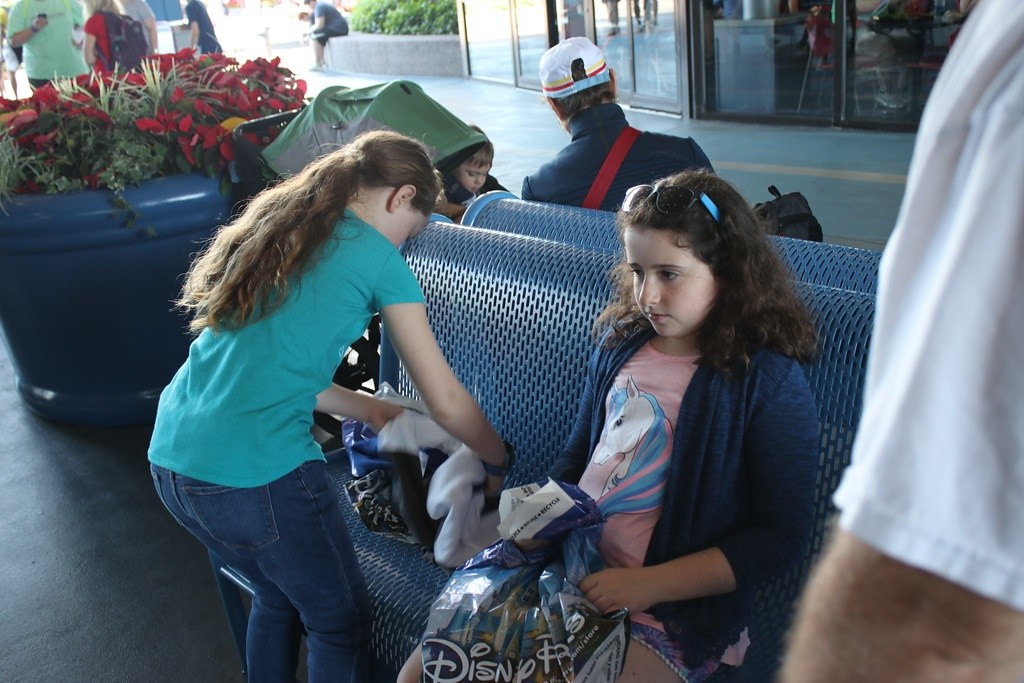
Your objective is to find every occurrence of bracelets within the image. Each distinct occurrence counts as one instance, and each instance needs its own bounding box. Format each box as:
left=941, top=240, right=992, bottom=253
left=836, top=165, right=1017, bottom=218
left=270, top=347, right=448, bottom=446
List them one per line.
left=30, top=24, right=38, bottom=34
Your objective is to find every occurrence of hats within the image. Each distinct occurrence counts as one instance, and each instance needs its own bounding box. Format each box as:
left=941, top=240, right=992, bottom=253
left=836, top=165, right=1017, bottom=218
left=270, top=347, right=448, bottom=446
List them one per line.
left=539, top=37, right=611, bottom=98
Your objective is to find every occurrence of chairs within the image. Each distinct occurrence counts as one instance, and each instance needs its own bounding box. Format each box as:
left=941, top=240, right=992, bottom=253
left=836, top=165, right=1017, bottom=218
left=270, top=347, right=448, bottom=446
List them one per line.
left=797, top=5, right=960, bottom=117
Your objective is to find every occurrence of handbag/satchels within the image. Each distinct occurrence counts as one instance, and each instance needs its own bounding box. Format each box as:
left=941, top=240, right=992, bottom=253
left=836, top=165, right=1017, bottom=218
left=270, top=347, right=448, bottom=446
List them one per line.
left=422, top=477, right=629, bottom=683
left=342, top=416, right=450, bottom=546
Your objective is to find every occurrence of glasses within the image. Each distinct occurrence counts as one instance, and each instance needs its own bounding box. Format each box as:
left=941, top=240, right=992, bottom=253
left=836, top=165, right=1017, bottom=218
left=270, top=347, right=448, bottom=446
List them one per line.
left=620, top=185, right=720, bottom=222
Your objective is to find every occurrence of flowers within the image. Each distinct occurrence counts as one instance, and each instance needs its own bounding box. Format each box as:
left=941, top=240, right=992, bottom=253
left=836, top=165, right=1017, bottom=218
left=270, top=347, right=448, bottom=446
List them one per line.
left=0, top=47, right=316, bottom=236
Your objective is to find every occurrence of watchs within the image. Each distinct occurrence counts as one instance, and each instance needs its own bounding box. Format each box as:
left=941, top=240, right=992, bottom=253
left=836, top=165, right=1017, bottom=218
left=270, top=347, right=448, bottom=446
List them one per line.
left=481, top=440, right=515, bottom=477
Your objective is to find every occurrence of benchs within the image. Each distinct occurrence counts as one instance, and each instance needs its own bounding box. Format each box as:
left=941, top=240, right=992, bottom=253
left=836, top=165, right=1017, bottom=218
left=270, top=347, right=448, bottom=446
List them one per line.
left=207, top=191, right=884, bottom=683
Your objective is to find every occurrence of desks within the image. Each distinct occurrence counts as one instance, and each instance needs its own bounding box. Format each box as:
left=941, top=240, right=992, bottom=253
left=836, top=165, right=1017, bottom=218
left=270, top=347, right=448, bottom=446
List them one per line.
left=871, top=16, right=966, bottom=117
left=714, top=11, right=810, bottom=62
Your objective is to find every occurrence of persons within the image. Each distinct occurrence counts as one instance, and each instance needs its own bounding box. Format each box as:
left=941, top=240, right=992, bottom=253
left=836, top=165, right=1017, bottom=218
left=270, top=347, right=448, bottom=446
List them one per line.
left=776, top=1, right=1024, bottom=683
left=147, top=129, right=508, bottom=683
left=0, top=0, right=225, bottom=100
left=393, top=172, right=817, bottom=683
left=298, top=0, right=349, bottom=71
left=518, top=36, right=718, bottom=212
left=428, top=140, right=510, bottom=222
left=600, top=0, right=658, bottom=36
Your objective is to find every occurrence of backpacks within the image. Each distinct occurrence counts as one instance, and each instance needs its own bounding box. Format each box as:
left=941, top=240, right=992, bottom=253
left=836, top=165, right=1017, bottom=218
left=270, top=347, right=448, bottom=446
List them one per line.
left=92, top=11, right=148, bottom=73
left=748, top=185, right=823, bottom=242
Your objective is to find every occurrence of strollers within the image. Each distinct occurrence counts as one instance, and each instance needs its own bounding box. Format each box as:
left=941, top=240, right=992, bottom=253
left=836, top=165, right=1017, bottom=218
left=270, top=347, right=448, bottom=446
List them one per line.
left=233, top=80, right=488, bottom=437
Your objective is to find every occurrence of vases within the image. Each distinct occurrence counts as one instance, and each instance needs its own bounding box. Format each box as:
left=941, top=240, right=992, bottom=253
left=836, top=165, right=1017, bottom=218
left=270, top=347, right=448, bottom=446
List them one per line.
left=0, top=174, right=253, bottom=424
